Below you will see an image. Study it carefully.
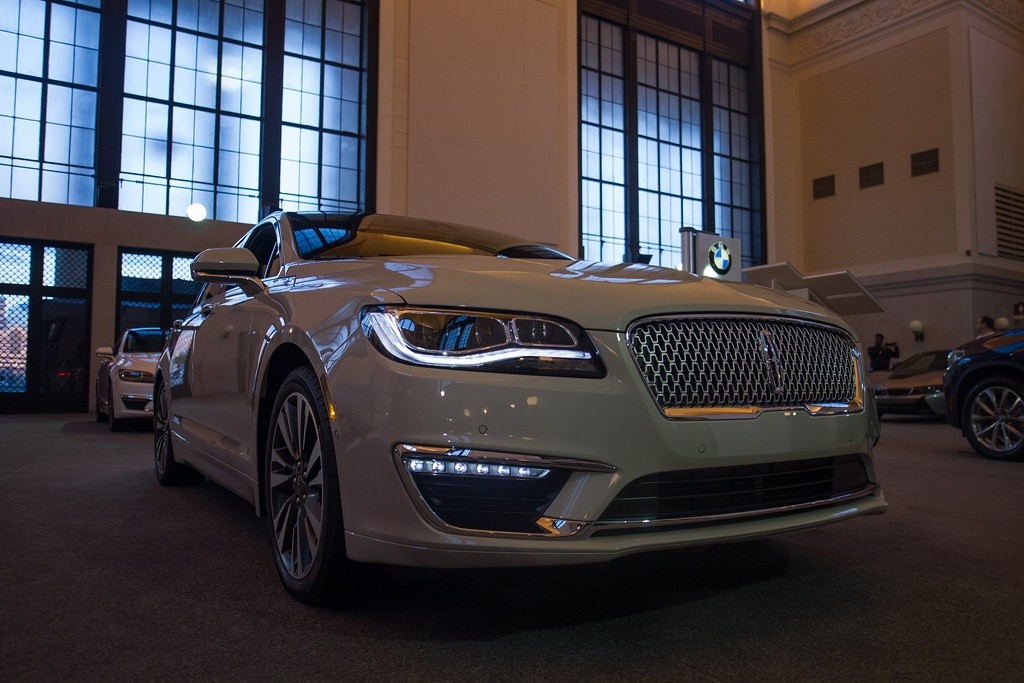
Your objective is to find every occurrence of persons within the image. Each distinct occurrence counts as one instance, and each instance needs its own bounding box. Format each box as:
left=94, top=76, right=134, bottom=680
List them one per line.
left=868, top=333, right=900, bottom=371
left=975, top=315, right=994, bottom=339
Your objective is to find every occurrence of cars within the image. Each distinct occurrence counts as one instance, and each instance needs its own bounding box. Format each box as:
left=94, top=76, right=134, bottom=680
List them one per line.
left=95, top=327, right=180, bottom=432
left=872, top=349, right=958, bottom=423
left=153, top=208, right=888, bottom=609
left=942, top=325, right=1024, bottom=461
